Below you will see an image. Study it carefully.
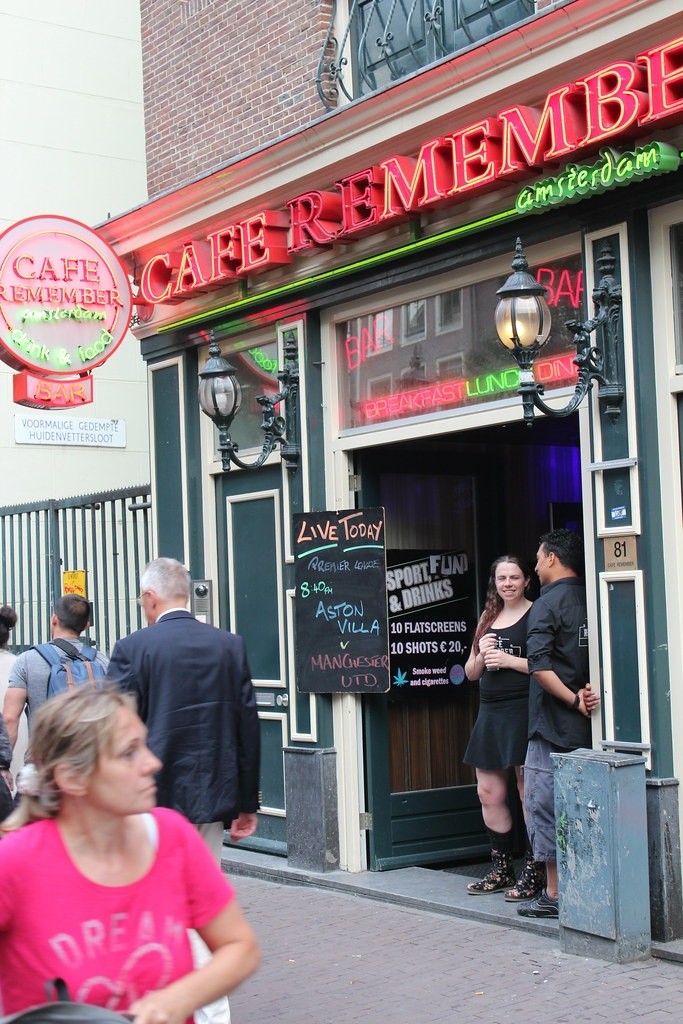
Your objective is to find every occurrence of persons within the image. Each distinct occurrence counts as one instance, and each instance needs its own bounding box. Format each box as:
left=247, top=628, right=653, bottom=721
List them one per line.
left=516, top=528, right=599, bottom=917
left=465, top=554, right=547, bottom=902
left=1, top=593, right=111, bottom=820
left=102, top=557, right=262, bottom=871
left=0, top=678, right=261, bottom=1024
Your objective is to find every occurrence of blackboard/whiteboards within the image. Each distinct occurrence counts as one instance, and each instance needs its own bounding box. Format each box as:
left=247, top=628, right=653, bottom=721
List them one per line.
left=291, top=506, right=391, bottom=694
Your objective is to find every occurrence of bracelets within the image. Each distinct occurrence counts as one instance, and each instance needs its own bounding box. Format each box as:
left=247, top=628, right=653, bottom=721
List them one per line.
left=569, top=694, right=580, bottom=710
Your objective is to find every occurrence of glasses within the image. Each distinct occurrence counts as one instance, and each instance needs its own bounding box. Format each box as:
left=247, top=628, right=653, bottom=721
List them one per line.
left=137, top=592, right=147, bottom=606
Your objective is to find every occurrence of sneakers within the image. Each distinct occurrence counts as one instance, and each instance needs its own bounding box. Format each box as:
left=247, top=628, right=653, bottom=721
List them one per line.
left=517, top=888, right=559, bottom=917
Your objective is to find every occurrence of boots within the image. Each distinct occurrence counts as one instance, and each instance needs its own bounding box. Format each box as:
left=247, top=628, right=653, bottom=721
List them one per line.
left=504, top=847, right=547, bottom=901
left=467, top=848, right=517, bottom=895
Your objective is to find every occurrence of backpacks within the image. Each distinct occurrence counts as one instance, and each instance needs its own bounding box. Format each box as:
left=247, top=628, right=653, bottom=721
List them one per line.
left=29, top=643, right=105, bottom=699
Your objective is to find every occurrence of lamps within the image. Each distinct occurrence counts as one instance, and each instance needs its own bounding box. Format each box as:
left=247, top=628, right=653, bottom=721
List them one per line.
left=494, top=236, right=623, bottom=428
left=197, top=327, right=299, bottom=472
